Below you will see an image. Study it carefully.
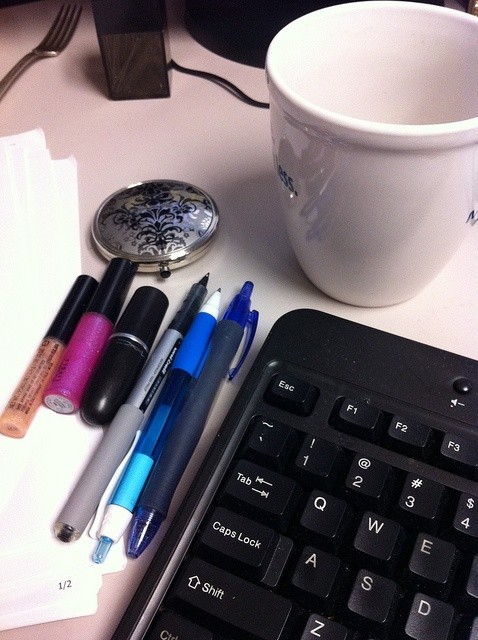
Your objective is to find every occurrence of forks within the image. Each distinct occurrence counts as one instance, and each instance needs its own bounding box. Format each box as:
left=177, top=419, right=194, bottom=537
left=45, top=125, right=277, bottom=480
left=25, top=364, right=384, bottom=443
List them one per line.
left=0, top=2, right=83, bottom=101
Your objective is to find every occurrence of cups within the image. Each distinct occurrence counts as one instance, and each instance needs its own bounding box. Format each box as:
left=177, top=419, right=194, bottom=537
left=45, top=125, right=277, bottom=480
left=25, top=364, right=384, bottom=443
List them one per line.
left=266, top=1, right=478, bottom=308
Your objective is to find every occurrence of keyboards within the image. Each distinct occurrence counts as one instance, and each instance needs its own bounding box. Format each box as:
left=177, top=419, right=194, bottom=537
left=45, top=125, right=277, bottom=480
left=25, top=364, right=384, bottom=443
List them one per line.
left=112, top=306, right=478, bottom=640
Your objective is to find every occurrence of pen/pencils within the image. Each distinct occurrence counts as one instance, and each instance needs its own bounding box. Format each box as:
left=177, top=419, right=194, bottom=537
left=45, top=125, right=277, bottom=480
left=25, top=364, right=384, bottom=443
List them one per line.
left=53, top=272, right=211, bottom=542
left=89, top=288, right=223, bottom=564
left=128, top=282, right=259, bottom=559
